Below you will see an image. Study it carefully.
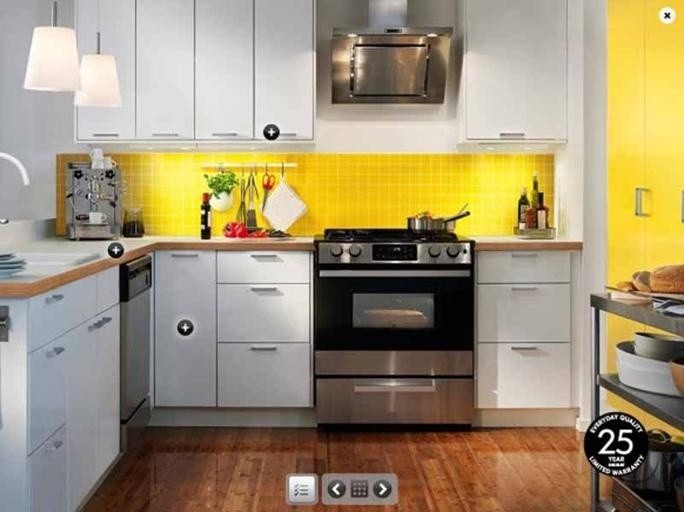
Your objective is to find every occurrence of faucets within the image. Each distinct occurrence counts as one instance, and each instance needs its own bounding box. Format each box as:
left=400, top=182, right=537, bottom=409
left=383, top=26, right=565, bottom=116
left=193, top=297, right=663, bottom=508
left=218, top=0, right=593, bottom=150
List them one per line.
left=0, top=152, right=31, bottom=186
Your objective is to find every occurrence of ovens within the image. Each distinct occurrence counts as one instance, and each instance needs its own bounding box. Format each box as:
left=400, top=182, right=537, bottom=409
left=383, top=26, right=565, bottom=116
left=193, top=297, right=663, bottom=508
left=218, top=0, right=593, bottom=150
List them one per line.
left=315, top=264, right=475, bottom=379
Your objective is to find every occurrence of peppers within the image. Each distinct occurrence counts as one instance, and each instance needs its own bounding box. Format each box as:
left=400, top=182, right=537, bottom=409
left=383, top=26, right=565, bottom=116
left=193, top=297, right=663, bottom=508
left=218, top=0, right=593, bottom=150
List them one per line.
left=222, top=221, right=249, bottom=239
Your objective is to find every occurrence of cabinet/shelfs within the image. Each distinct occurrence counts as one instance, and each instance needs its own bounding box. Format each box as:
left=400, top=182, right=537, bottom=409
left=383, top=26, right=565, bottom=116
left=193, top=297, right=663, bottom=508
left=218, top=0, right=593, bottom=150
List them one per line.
left=1, top=262, right=120, bottom=511
left=148, top=246, right=218, bottom=408
left=468, top=235, right=582, bottom=410
left=212, top=240, right=312, bottom=409
left=134, top=0, right=316, bottom=145
left=587, top=286, right=682, bottom=512
left=453, top=2, right=569, bottom=148
left=601, top=1, right=682, bottom=440
left=69, top=0, right=133, bottom=143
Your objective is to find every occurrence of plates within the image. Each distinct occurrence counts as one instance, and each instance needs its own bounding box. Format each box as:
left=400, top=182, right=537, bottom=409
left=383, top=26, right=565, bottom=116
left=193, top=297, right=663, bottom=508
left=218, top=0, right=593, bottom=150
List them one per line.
left=0, top=251, right=27, bottom=279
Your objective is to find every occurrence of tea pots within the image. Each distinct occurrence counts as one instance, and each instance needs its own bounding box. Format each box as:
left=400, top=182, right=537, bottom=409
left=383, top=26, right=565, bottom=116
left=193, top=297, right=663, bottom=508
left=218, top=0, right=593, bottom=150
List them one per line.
left=122, top=207, right=144, bottom=237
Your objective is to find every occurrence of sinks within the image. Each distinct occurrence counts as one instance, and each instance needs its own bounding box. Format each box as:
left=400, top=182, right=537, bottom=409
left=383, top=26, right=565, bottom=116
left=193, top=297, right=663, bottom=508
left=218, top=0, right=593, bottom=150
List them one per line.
left=15, top=252, right=100, bottom=266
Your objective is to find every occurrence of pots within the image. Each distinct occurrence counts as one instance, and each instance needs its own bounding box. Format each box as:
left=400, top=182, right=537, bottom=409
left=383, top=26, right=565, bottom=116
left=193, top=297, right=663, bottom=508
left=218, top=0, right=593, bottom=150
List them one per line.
left=406, top=210, right=470, bottom=232
left=616, top=428, right=683, bottom=510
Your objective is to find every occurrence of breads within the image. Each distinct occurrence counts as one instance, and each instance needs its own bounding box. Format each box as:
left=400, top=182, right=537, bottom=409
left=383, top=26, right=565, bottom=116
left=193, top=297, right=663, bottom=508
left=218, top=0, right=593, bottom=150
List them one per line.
left=630, top=264, right=684, bottom=294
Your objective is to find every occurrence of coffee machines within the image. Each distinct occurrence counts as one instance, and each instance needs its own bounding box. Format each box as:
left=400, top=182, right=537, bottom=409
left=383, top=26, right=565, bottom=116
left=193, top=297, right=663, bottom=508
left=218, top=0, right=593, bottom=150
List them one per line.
left=62, top=159, right=120, bottom=239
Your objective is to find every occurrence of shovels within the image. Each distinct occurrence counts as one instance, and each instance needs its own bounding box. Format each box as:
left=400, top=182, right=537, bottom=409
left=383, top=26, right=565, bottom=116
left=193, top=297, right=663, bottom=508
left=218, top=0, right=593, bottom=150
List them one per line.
left=247, top=175, right=256, bottom=227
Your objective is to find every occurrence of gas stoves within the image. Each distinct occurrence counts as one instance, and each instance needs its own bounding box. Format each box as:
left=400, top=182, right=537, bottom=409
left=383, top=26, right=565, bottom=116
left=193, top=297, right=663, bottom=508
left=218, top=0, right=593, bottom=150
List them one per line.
left=327, top=229, right=456, bottom=241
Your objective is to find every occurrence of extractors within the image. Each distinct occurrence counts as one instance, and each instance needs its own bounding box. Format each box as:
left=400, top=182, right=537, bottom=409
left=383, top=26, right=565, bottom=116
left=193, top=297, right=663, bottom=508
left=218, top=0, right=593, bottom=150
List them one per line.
left=330, top=1, right=452, bottom=106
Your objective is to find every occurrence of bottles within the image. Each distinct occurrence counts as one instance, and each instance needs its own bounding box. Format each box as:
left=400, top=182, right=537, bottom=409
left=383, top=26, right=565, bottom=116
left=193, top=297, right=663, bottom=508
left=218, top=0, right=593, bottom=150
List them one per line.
left=199, top=192, right=211, bottom=239
left=517, top=176, right=548, bottom=229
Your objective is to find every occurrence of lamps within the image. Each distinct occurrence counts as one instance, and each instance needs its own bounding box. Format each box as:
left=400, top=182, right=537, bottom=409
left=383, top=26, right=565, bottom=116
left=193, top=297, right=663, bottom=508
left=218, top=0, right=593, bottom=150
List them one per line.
left=20, top=0, right=82, bottom=94
left=73, top=2, right=124, bottom=105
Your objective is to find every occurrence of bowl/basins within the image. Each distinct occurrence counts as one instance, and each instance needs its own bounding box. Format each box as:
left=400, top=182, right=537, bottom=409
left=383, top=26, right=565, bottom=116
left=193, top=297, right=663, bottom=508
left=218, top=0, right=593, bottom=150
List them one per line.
left=613, top=332, right=684, bottom=399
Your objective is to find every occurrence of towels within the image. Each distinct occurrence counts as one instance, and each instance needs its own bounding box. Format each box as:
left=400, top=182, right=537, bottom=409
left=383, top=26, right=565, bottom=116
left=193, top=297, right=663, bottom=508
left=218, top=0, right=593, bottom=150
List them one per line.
left=258, top=179, right=309, bottom=234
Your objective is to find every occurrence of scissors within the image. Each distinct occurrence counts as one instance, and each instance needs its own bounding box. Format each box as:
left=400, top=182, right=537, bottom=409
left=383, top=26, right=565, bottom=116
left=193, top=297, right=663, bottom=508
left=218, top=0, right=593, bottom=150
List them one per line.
left=262, top=175, right=276, bottom=214
